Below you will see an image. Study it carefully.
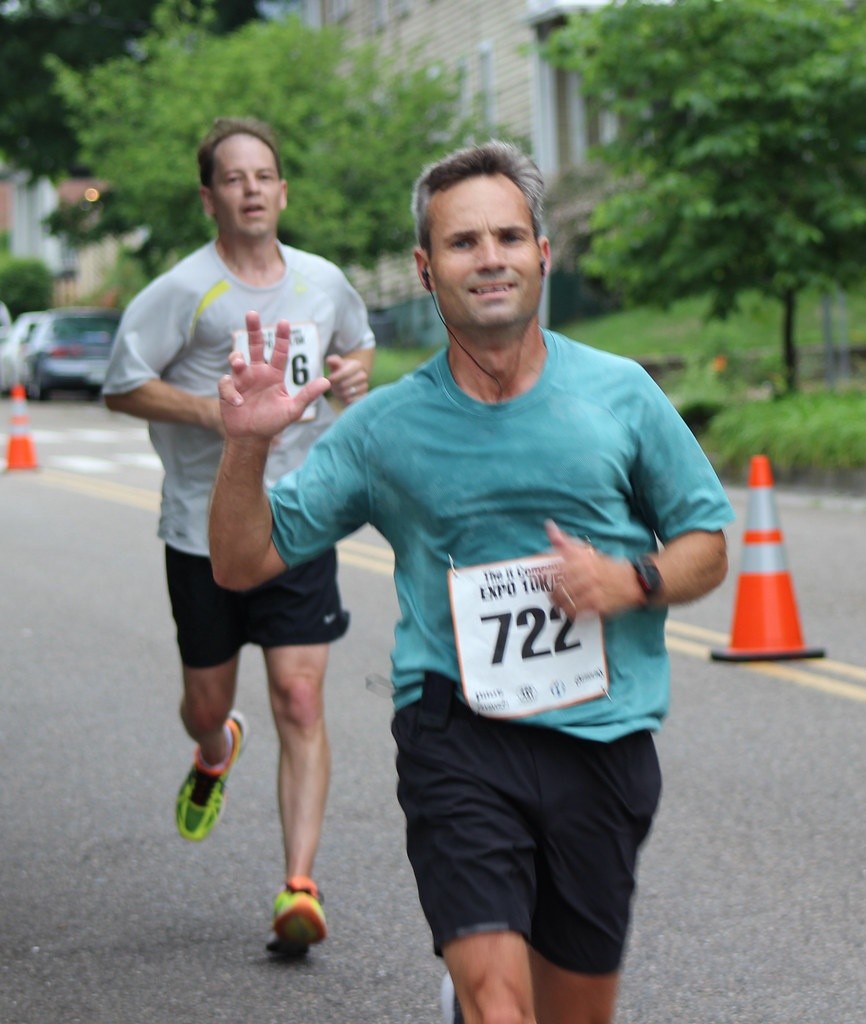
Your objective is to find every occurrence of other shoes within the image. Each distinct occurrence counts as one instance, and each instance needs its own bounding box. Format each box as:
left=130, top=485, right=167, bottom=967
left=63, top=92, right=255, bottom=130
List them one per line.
left=439, top=971, right=464, bottom=1024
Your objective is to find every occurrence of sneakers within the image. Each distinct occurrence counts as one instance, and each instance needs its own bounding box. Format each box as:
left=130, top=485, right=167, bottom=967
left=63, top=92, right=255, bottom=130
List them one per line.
left=266, top=874, right=327, bottom=961
left=175, top=708, right=247, bottom=839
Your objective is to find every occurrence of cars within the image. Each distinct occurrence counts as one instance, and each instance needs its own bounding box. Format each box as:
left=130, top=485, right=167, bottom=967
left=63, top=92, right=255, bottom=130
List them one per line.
left=0, top=301, right=122, bottom=399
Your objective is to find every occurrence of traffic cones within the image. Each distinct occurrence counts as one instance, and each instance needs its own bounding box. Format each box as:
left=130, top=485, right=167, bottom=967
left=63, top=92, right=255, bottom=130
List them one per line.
left=707, top=455, right=830, bottom=662
left=3, top=384, right=42, bottom=471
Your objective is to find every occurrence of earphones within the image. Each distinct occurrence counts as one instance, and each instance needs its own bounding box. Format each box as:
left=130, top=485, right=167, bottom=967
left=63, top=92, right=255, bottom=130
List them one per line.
left=540, top=257, right=545, bottom=277
left=421, top=263, right=432, bottom=290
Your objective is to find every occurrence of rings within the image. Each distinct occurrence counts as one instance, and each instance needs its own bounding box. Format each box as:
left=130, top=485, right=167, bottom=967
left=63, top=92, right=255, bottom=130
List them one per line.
left=560, top=586, right=577, bottom=608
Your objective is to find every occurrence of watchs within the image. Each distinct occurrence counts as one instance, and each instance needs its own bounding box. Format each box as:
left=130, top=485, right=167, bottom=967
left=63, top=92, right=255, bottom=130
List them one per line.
left=631, top=553, right=664, bottom=610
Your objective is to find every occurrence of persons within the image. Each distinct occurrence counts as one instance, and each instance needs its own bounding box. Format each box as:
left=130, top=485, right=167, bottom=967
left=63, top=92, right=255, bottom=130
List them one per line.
left=100, top=115, right=375, bottom=964
left=207, top=141, right=737, bottom=1023
